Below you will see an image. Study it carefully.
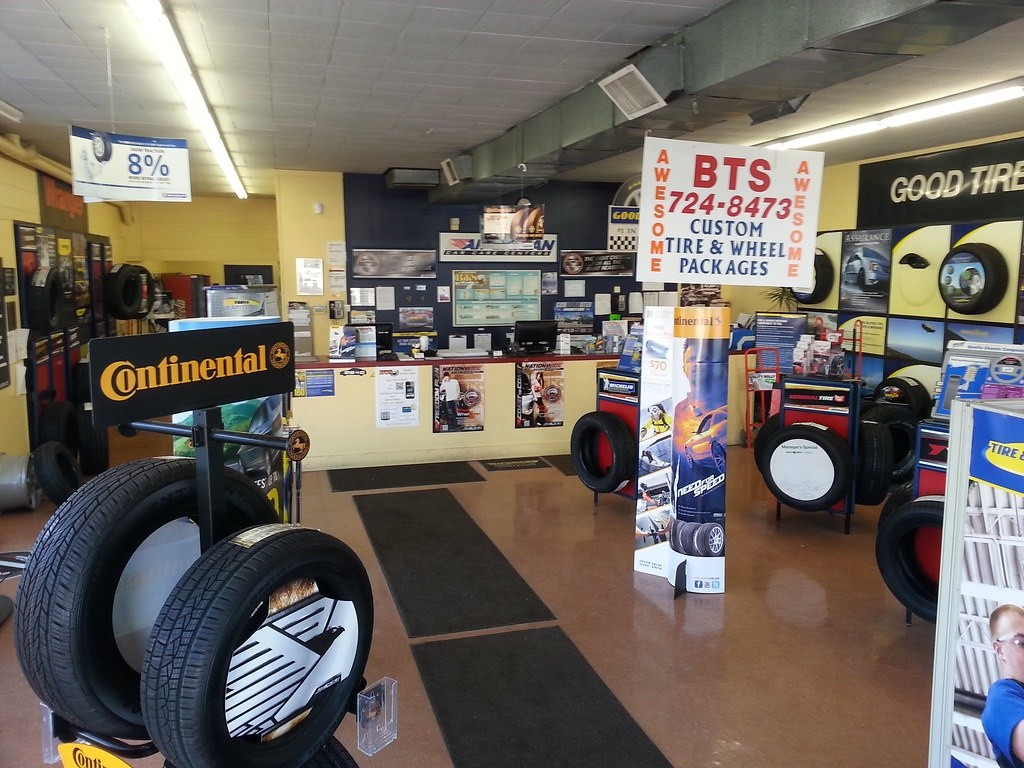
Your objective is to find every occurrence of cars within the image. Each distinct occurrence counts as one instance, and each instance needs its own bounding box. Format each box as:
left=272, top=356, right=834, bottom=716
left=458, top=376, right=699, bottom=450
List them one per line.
left=683, top=405, right=726, bottom=478
left=843, top=247, right=891, bottom=295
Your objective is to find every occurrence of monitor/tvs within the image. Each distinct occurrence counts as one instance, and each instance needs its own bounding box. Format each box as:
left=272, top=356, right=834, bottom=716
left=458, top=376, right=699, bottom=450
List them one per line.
left=514, top=321, right=557, bottom=354
left=343, top=322, right=394, bottom=354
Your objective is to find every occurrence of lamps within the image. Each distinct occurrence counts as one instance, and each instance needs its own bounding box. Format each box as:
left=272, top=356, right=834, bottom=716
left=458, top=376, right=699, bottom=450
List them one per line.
left=124, top=1, right=248, bottom=200
left=754, top=74, right=1024, bottom=149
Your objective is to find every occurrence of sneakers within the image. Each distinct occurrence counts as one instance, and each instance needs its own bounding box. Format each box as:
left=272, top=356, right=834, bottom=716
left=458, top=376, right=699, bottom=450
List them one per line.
left=537, top=422, right=541, bottom=425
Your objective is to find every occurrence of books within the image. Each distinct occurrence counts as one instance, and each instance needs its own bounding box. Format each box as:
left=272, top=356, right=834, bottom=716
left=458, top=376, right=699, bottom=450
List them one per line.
left=791, top=333, right=831, bottom=379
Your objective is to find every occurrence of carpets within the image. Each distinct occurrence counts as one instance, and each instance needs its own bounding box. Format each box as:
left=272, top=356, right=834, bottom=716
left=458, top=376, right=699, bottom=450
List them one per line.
left=326, top=460, right=487, bottom=493
left=478, top=456, right=552, bottom=471
left=352, top=488, right=559, bottom=639
left=409, top=627, right=674, bottom=768
left=541, top=454, right=578, bottom=476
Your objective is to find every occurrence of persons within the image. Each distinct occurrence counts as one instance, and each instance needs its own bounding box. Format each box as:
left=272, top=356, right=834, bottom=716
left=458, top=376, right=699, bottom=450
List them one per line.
left=981, top=604, right=1024, bottom=768
left=522, top=371, right=546, bottom=426
left=636, top=484, right=671, bottom=539
left=642, top=450, right=657, bottom=464
left=641, top=403, right=672, bottom=437
left=439, top=372, right=461, bottom=429
left=668, top=335, right=723, bottom=519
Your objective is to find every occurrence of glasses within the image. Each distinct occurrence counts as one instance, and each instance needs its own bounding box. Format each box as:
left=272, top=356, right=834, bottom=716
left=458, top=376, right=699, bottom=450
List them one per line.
left=997, top=637, right=1024, bottom=649
